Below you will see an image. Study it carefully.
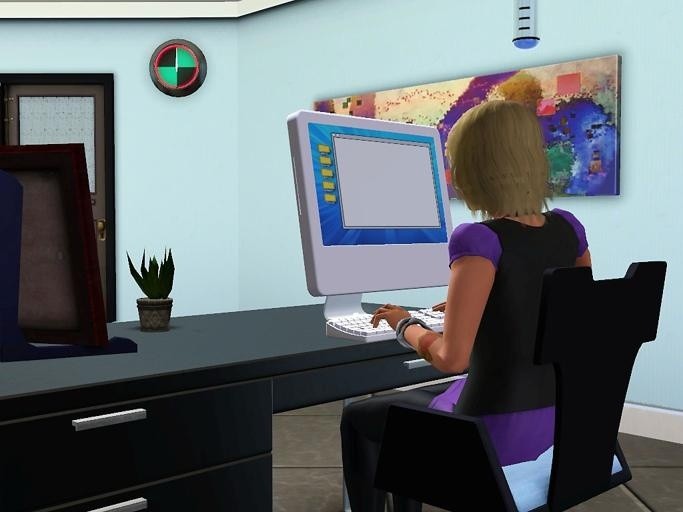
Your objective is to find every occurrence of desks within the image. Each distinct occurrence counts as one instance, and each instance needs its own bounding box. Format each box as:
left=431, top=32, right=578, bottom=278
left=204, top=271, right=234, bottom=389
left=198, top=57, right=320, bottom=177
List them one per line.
left=0, top=300, right=472, bottom=512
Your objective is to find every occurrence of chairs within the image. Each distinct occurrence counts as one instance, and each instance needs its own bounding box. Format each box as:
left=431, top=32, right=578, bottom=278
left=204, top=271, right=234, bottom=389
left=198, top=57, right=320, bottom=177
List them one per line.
left=360, top=257, right=666, bottom=512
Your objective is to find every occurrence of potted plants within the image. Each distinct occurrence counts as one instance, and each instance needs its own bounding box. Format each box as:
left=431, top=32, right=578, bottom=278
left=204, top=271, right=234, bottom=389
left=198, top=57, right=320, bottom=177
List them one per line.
left=122, top=245, right=178, bottom=331
